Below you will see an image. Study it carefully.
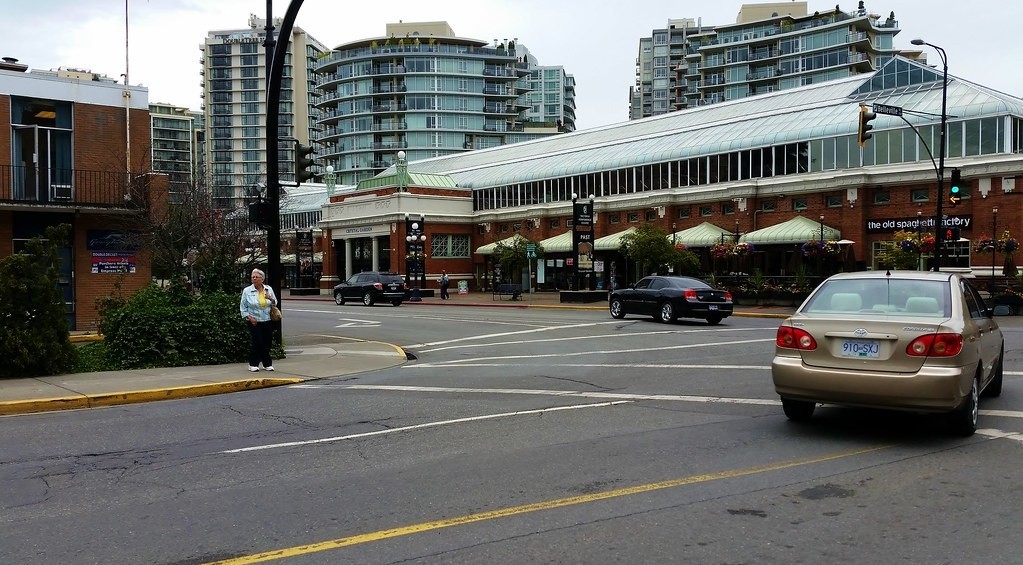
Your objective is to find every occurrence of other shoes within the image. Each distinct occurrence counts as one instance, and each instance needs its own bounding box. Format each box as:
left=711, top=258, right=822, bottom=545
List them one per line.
left=447, top=294, right=450, bottom=299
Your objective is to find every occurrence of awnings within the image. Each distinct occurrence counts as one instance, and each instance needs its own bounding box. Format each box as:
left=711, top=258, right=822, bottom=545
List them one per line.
left=235, top=251, right=323, bottom=264
left=473, top=216, right=841, bottom=254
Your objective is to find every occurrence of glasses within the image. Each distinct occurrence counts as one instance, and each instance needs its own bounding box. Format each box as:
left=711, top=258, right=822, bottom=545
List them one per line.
left=252, top=276, right=262, bottom=279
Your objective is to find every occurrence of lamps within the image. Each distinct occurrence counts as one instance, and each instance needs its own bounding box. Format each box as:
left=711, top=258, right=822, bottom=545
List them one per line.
left=395, top=152, right=408, bottom=192
left=324, top=166, right=336, bottom=203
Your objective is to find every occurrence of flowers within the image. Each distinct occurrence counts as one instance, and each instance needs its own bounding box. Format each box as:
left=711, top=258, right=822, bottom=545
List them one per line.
left=973, top=231, right=1020, bottom=256
left=901, top=237, right=935, bottom=252
left=674, top=243, right=686, bottom=250
left=710, top=241, right=755, bottom=259
left=802, top=240, right=841, bottom=257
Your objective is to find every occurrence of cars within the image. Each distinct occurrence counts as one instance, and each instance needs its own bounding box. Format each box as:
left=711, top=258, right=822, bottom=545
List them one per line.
left=333, top=272, right=409, bottom=307
left=770, top=268, right=1005, bottom=437
left=609, top=276, right=733, bottom=326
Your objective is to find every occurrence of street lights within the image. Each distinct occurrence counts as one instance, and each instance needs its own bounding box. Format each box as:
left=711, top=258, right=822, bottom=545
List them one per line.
left=911, top=39, right=948, bottom=273
left=672, top=222, right=677, bottom=245
left=735, top=219, right=740, bottom=248
left=406, top=221, right=427, bottom=301
left=993, top=206, right=998, bottom=298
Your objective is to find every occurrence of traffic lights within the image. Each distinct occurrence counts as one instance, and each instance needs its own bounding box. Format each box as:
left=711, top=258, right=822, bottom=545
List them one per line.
left=249, top=201, right=265, bottom=223
left=858, top=107, right=877, bottom=148
left=294, top=143, right=314, bottom=183
left=950, top=170, right=961, bottom=204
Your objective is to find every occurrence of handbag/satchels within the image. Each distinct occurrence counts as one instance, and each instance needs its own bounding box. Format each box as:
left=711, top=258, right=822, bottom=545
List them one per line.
left=270, top=304, right=282, bottom=321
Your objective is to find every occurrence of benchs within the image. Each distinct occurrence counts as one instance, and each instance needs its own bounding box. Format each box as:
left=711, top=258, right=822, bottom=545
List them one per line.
left=493, top=284, right=523, bottom=301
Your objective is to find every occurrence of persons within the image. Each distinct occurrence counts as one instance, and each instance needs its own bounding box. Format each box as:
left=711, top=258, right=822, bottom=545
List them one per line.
left=240, top=268, right=277, bottom=371
left=437, top=270, right=450, bottom=299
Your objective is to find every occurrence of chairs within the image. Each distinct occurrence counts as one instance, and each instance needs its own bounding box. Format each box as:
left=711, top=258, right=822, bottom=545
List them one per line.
left=905, top=297, right=939, bottom=314
left=830, top=292, right=863, bottom=311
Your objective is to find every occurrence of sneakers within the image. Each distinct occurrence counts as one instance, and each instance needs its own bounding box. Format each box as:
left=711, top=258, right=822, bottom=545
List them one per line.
left=248, top=365, right=260, bottom=372
left=263, top=365, right=275, bottom=371
